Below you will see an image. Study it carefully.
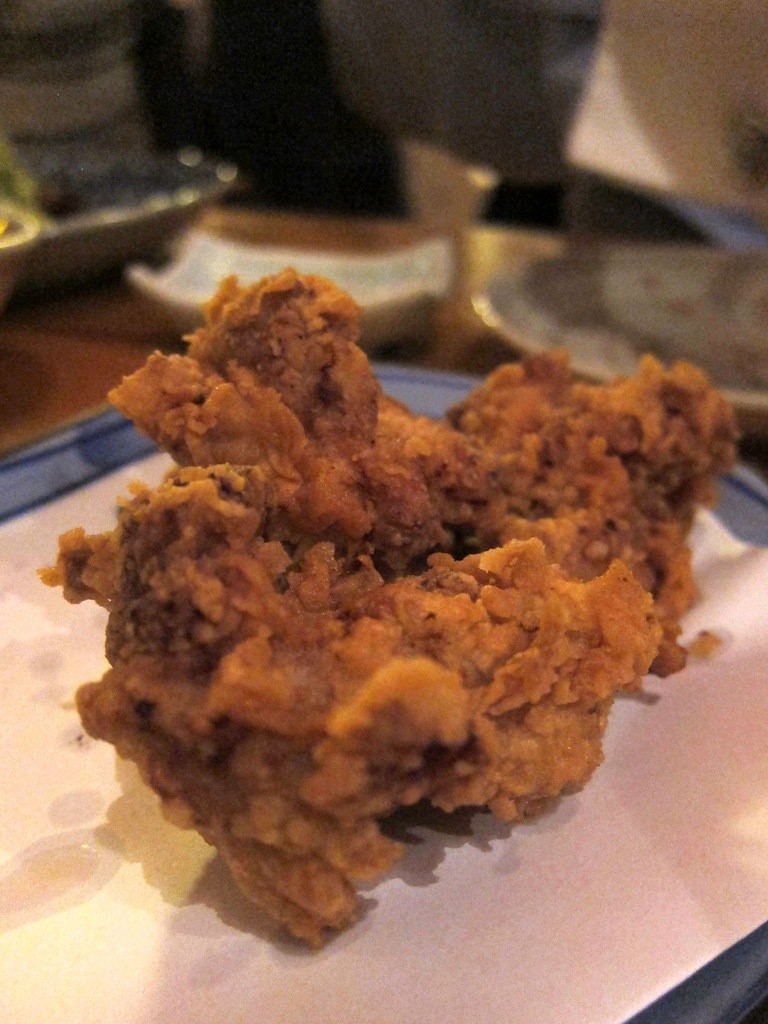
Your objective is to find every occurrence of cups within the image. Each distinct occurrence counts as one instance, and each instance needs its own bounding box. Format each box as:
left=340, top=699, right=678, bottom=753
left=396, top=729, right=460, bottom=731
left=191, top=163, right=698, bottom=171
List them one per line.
left=0, top=0, right=157, bottom=218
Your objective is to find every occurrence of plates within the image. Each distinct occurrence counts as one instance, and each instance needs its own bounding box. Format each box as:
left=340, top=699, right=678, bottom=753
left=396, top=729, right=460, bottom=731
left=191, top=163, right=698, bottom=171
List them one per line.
left=477, top=236, right=768, bottom=447
left=126, top=233, right=452, bottom=347
left=0, top=356, right=768, bottom=1024
